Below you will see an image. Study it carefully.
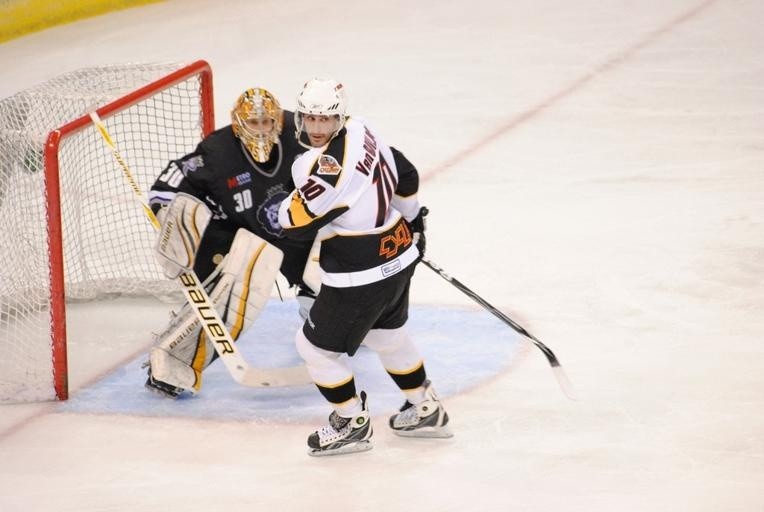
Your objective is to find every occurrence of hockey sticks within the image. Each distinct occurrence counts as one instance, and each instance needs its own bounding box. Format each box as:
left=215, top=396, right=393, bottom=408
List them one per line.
left=88, top=110, right=312, bottom=387
left=421, top=255, right=582, bottom=401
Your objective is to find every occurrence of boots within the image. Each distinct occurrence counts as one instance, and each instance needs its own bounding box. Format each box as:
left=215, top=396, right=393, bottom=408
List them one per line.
left=307, top=390, right=372, bottom=450
left=389, top=380, right=449, bottom=432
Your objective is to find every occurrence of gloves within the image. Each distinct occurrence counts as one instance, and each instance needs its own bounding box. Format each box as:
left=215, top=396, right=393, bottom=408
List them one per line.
left=405, top=205, right=428, bottom=259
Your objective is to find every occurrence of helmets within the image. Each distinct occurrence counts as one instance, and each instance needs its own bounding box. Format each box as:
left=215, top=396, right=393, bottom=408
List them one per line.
left=295, top=78, right=351, bottom=137
left=231, top=88, right=284, bottom=164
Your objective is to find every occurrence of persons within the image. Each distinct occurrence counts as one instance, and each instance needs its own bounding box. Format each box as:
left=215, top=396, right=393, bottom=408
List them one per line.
left=144, top=88, right=310, bottom=397
left=266, top=76, right=452, bottom=455
